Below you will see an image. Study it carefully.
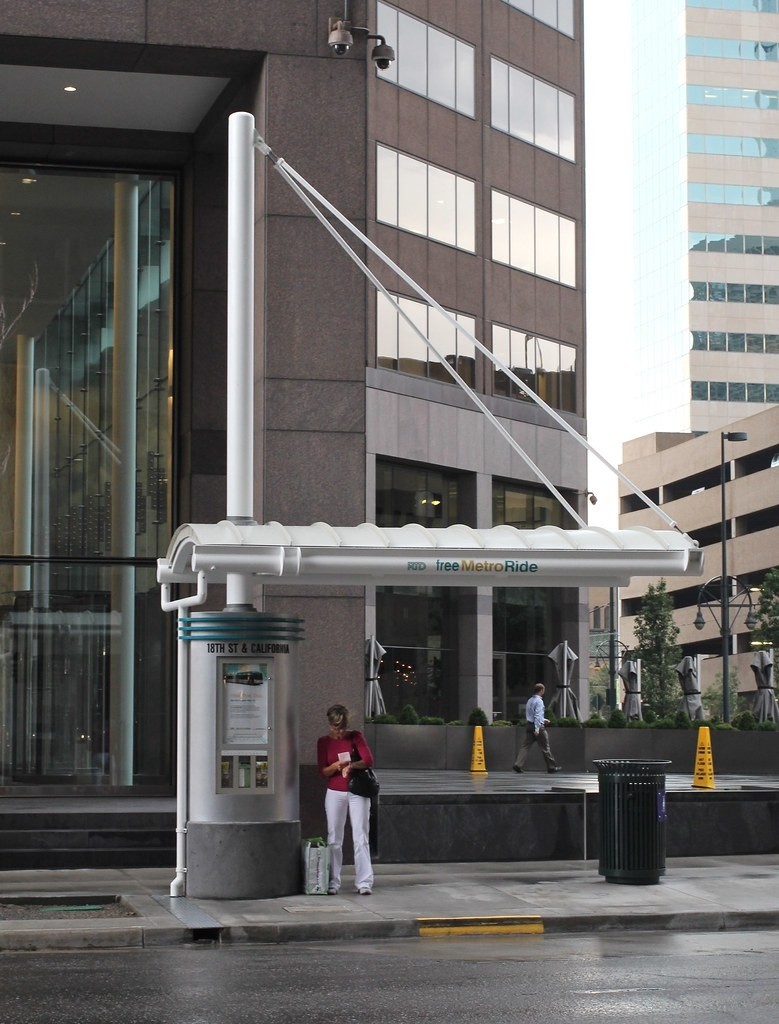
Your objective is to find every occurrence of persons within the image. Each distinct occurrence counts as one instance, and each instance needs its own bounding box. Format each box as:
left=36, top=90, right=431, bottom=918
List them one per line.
left=317, top=705, right=374, bottom=894
left=513, top=683, right=562, bottom=772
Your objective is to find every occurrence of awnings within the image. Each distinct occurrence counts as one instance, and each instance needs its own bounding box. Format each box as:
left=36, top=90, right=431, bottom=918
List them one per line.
left=165, top=521, right=705, bottom=587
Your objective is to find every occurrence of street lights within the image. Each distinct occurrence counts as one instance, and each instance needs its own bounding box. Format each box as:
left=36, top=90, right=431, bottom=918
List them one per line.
left=693, top=431, right=761, bottom=726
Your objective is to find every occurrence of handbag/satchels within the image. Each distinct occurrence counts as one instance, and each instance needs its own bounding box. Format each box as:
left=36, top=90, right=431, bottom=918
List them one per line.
left=300, top=836, right=330, bottom=895
left=348, top=731, right=380, bottom=798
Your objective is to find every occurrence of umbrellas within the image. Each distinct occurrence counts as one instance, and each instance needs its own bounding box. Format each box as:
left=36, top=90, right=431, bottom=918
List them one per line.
left=365, top=637, right=779, bottom=724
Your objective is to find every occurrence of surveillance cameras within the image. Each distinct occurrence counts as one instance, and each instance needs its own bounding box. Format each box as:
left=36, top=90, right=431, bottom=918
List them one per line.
left=327, top=30, right=353, bottom=55
left=371, top=45, right=396, bottom=69
left=589, top=496, right=597, bottom=505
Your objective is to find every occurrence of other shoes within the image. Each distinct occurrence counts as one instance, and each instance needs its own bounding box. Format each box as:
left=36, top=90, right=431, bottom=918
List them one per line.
left=328, top=886, right=337, bottom=895
left=358, top=887, right=372, bottom=895
left=513, top=765, right=525, bottom=773
left=547, top=766, right=562, bottom=774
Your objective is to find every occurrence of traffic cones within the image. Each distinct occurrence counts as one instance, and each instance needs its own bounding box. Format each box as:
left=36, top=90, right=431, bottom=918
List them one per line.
left=469, top=725, right=487, bottom=771
left=691, top=726, right=716, bottom=789
left=469, top=771, right=488, bottom=791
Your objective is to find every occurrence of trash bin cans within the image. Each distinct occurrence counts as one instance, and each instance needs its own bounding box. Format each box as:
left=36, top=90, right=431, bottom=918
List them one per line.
left=593, top=758, right=671, bottom=887
